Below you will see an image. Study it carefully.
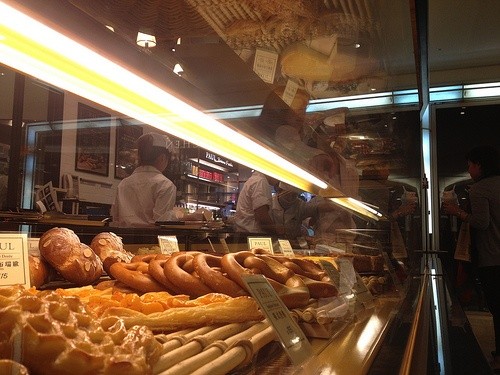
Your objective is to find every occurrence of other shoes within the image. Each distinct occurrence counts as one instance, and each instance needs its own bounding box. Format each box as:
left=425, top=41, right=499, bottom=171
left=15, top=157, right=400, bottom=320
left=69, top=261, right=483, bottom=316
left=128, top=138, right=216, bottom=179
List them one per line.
left=492, top=368, right=500, bottom=374
left=491, top=351, right=496, bottom=355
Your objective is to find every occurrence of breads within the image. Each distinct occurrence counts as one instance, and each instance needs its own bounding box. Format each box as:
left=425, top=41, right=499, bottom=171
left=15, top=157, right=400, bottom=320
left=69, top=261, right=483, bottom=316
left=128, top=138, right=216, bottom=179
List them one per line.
left=0, top=227, right=390, bottom=375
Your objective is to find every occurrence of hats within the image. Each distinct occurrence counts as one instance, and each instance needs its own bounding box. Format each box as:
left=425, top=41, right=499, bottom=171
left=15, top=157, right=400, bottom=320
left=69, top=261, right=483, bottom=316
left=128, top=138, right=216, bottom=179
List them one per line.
left=137, top=132, right=178, bottom=153
left=278, top=181, right=304, bottom=195
left=276, top=124, right=301, bottom=152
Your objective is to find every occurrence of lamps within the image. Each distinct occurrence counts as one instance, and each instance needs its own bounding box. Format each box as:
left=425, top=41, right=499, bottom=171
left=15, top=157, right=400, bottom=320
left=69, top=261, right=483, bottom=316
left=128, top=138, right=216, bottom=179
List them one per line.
left=0, top=0, right=383, bottom=223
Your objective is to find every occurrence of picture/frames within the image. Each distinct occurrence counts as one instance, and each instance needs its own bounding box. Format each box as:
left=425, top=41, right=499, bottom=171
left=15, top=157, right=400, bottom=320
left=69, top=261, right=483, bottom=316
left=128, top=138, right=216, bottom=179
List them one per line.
left=114, top=117, right=144, bottom=181
left=74, top=102, right=109, bottom=176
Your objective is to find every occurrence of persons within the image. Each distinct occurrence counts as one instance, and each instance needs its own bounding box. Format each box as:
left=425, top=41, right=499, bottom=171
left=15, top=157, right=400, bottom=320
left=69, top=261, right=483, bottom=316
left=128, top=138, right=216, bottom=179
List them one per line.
left=110, top=132, right=209, bottom=228
left=442, top=141, right=500, bottom=360
left=232, top=171, right=357, bottom=248
left=358, top=159, right=417, bottom=261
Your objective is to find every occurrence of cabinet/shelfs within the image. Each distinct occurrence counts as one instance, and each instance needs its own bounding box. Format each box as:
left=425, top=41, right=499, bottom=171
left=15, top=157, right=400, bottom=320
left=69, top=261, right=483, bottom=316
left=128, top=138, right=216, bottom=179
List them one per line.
left=175, top=145, right=242, bottom=220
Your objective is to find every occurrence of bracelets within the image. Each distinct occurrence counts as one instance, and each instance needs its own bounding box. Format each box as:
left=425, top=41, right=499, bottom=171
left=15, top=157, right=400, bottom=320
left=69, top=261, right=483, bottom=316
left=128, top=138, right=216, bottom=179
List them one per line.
left=456, top=209, right=462, bottom=217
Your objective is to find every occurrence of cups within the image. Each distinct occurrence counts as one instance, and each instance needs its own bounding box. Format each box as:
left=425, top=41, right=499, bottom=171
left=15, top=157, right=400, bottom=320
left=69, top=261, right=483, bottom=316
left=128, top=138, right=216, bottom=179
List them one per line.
left=403, top=192, right=416, bottom=213
left=443, top=190, right=454, bottom=202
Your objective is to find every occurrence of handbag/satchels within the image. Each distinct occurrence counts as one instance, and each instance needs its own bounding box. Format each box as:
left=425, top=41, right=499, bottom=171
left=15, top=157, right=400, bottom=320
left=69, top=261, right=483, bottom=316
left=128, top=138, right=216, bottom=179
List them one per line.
left=389, top=220, right=408, bottom=259
left=453, top=221, right=472, bottom=262
